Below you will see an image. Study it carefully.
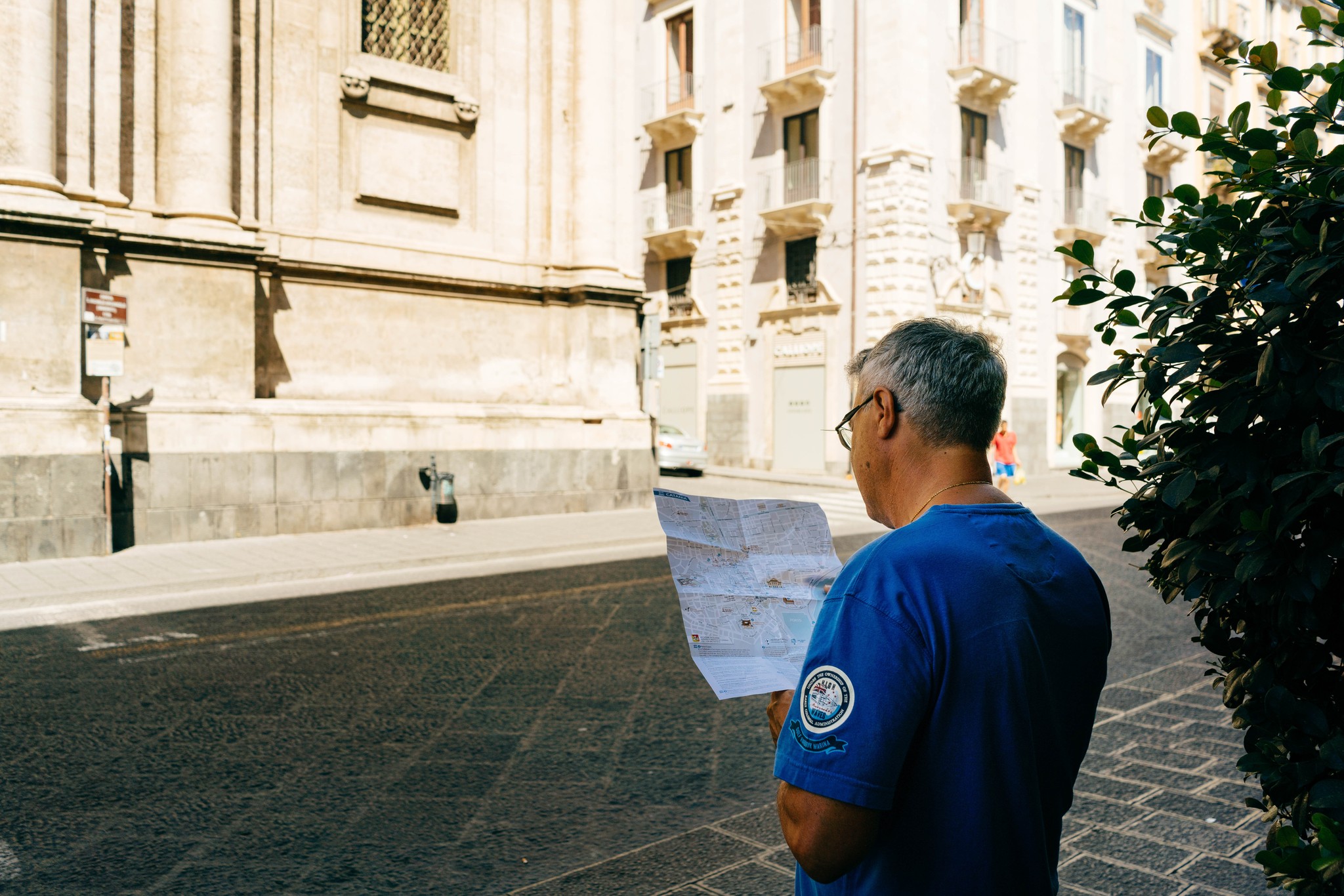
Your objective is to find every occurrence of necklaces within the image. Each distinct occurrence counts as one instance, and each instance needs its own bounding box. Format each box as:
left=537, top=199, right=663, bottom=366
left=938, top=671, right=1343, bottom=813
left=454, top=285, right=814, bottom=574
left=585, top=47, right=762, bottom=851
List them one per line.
left=910, top=482, right=993, bottom=524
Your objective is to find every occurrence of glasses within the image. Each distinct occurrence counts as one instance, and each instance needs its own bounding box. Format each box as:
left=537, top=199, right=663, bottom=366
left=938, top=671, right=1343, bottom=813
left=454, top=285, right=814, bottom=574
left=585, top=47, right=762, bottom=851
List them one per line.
left=835, top=391, right=874, bottom=453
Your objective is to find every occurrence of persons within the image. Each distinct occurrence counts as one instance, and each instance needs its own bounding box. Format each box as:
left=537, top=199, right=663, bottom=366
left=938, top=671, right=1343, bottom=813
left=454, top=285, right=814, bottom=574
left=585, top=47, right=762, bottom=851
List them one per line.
left=992, top=419, right=1022, bottom=493
left=766, top=318, right=1112, bottom=896
